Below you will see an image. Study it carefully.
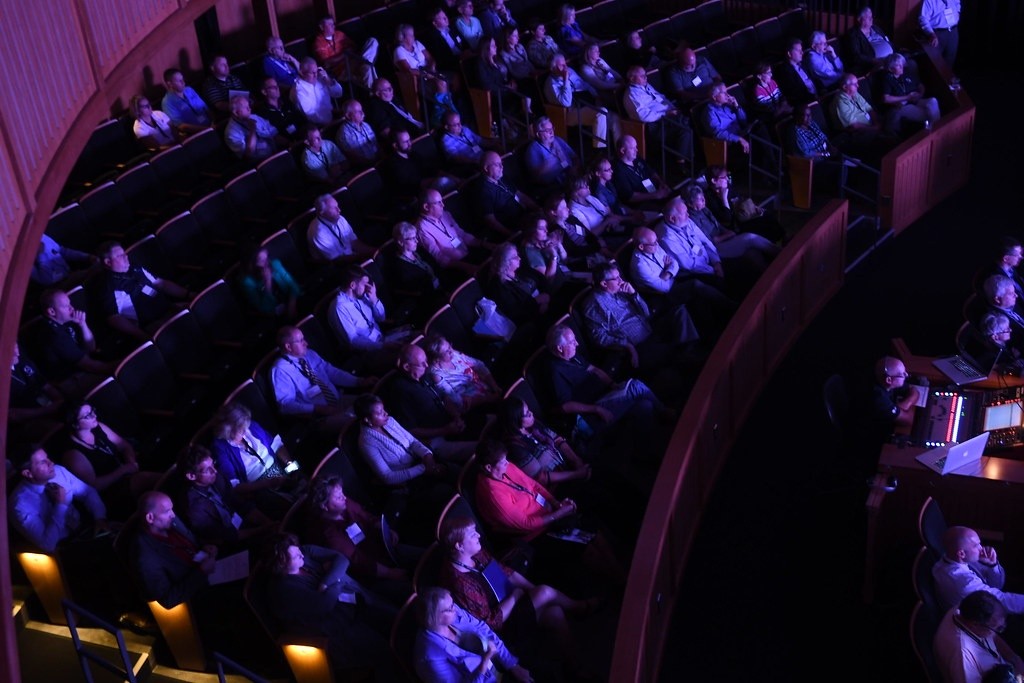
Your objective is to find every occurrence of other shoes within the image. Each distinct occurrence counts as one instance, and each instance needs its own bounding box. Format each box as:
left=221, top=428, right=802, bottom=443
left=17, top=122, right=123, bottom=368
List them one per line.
left=673, top=167, right=690, bottom=178
left=575, top=594, right=609, bottom=623
left=564, top=666, right=601, bottom=683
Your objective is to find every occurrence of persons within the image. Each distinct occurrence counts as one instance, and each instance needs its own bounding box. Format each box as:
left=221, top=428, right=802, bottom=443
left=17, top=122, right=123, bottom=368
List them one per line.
left=3, top=0, right=1024, bottom=683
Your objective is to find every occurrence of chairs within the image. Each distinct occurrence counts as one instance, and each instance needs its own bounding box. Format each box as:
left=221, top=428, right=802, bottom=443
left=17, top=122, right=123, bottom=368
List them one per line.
left=913, top=48, right=944, bottom=98
left=865, top=57, right=924, bottom=129
left=776, top=52, right=810, bottom=95
left=830, top=76, right=891, bottom=143
left=6, top=0, right=773, bottom=683
left=806, top=37, right=848, bottom=87
left=821, top=374, right=846, bottom=430
left=842, top=23, right=887, bottom=71
left=775, top=100, right=844, bottom=175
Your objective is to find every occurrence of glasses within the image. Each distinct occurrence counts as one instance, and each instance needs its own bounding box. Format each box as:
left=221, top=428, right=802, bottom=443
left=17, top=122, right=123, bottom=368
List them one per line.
left=605, top=273, right=623, bottom=281
left=539, top=126, right=555, bottom=133
left=440, top=600, right=455, bottom=613
left=78, top=406, right=96, bottom=421
left=891, top=371, right=909, bottom=379
left=579, top=184, right=590, bottom=190
left=403, top=235, right=418, bottom=240
left=140, top=103, right=152, bottom=108
left=198, top=459, right=217, bottom=473
left=290, top=336, right=305, bottom=344
left=643, top=235, right=659, bottom=246
left=449, top=121, right=461, bottom=127
left=981, top=623, right=1007, bottom=634
left=719, top=175, right=728, bottom=180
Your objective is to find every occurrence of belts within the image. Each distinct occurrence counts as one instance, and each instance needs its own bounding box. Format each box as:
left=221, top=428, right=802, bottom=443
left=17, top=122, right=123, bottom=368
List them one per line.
left=934, top=24, right=958, bottom=32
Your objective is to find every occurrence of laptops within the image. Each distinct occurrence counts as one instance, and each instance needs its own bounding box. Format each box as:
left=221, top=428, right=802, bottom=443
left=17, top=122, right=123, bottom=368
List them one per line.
left=931, top=323, right=1002, bottom=385
left=915, top=432, right=990, bottom=476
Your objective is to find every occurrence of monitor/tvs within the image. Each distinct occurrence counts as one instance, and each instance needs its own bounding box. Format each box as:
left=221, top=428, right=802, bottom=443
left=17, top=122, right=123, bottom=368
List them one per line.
left=983, top=400, right=1023, bottom=431
